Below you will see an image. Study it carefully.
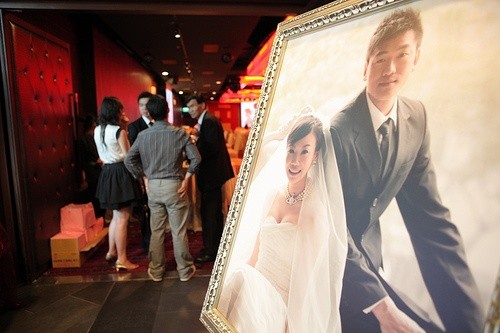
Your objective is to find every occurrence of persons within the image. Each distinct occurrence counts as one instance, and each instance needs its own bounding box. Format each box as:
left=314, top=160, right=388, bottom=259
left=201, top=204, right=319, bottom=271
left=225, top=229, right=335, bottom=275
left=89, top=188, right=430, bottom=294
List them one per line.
left=215, top=115, right=347, bottom=333
left=187, top=97, right=235, bottom=265
left=93, top=96, right=140, bottom=271
left=127, top=92, right=156, bottom=255
left=76, top=114, right=103, bottom=196
left=329, top=10, right=488, bottom=333
left=124, top=95, right=201, bottom=282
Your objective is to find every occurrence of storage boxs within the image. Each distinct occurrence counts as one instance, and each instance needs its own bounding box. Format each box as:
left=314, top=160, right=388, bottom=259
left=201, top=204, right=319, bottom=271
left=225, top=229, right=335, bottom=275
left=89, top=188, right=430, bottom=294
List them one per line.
left=50, top=202, right=109, bottom=268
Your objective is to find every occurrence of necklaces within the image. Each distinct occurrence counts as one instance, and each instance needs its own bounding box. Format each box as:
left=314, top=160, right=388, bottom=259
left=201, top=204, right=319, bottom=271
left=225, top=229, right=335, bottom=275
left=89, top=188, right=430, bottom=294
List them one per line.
left=282, top=178, right=313, bottom=205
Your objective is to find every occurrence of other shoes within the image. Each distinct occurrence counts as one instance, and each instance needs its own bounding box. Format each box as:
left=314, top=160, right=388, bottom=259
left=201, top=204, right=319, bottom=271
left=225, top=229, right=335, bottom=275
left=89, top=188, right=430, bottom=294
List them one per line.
left=180, top=265, right=196, bottom=282
left=147, top=268, right=162, bottom=281
left=195, top=254, right=213, bottom=263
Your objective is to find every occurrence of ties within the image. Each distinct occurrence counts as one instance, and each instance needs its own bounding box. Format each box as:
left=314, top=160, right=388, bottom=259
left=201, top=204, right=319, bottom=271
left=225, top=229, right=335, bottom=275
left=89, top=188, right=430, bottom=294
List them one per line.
left=150, top=122, right=153, bottom=126
left=379, top=119, right=395, bottom=180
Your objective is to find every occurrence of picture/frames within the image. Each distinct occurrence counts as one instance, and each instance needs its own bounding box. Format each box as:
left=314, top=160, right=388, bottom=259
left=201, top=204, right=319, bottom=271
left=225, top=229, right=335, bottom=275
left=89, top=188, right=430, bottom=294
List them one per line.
left=200, top=0, right=500, bottom=333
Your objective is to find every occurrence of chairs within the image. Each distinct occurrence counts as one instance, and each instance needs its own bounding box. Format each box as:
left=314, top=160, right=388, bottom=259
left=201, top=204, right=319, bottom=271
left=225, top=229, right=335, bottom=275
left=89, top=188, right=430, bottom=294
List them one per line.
left=221, top=124, right=250, bottom=173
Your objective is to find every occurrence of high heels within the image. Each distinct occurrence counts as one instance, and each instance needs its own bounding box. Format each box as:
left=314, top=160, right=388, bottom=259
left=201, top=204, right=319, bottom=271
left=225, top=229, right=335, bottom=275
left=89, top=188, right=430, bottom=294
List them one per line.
left=115, top=258, right=139, bottom=271
left=105, top=253, right=117, bottom=263
